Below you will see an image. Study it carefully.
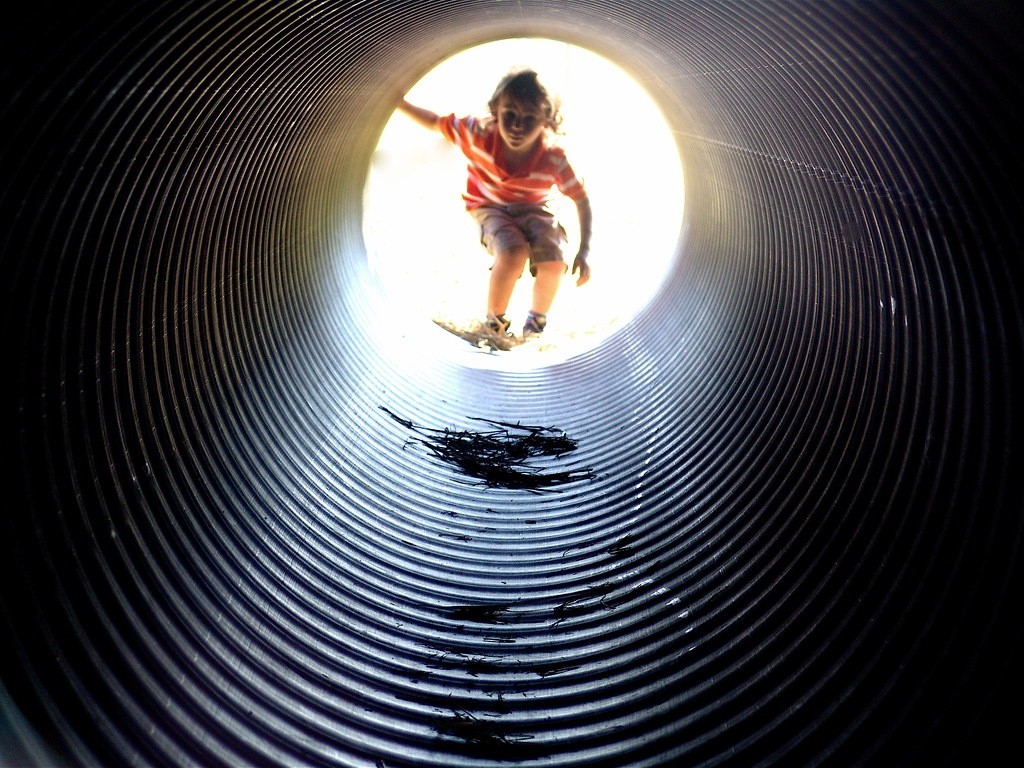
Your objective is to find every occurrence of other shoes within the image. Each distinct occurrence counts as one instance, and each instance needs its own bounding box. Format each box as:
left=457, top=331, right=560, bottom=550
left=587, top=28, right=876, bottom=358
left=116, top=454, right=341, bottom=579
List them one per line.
left=524, top=310, right=546, bottom=342
left=485, top=314, right=518, bottom=347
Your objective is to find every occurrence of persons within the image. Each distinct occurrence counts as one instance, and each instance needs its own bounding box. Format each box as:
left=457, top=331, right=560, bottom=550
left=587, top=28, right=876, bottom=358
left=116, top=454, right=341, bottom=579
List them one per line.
left=396, top=71, right=592, bottom=339
